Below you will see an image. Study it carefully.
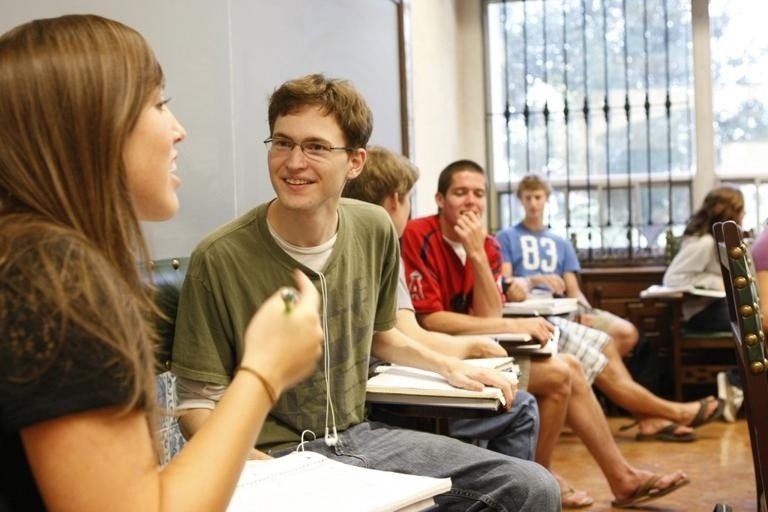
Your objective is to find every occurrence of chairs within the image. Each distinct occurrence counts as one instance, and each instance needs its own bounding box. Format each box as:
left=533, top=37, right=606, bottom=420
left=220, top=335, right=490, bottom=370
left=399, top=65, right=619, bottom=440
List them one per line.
left=669, top=294, right=738, bottom=411
left=711, top=222, right=767, bottom=509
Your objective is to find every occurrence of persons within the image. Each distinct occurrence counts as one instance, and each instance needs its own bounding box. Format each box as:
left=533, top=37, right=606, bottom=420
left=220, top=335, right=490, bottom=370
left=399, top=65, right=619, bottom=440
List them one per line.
left=496, top=174, right=726, bottom=442
left=170, top=75, right=561, bottom=512
left=0, top=14, right=324, bottom=512
left=342, top=147, right=541, bottom=461
left=662, top=187, right=757, bottom=423
left=402, top=160, right=690, bottom=508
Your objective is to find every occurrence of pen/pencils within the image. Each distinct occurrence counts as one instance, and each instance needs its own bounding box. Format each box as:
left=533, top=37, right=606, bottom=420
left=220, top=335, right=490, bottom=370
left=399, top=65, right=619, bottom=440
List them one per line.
left=280, top=285, right=296, bottom=313
left=534, top=311, right=554, bottom=342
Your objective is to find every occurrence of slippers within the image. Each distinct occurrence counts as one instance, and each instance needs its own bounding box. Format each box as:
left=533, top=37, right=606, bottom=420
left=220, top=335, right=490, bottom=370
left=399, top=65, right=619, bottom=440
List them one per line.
left=562, top=488, right=594, bottom=508
left=636, top=398, right=726, bottom=441
left=611, top=470, right=691, bottom=509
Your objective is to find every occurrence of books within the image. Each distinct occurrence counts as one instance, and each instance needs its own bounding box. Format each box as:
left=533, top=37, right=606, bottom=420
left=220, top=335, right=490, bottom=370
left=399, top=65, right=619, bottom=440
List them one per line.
left=503, top=297, right=579, bottom=316
left=487, top=326, right=560, bottom=358
left=226, top=450, right=452, bottom=512
left=365, top=356, right=521, bottom=412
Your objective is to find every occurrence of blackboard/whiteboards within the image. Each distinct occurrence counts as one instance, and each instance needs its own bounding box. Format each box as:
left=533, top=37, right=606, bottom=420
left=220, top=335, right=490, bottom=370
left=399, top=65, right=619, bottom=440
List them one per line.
left=0, top=0, right=410, bottom=269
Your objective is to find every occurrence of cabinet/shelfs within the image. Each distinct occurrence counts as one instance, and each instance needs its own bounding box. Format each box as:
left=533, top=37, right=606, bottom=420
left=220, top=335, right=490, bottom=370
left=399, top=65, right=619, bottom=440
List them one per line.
left=596, top=279, right=670, bottom=374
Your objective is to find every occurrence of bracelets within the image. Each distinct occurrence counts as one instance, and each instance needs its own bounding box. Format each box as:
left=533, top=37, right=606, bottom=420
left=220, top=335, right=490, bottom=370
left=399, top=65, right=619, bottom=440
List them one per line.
left=233, top=365, right=277, bottom=409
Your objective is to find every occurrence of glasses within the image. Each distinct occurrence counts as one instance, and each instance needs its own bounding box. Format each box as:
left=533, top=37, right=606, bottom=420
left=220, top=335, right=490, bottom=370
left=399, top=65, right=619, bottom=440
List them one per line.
left=263, top=137, right=351, bottom=163
